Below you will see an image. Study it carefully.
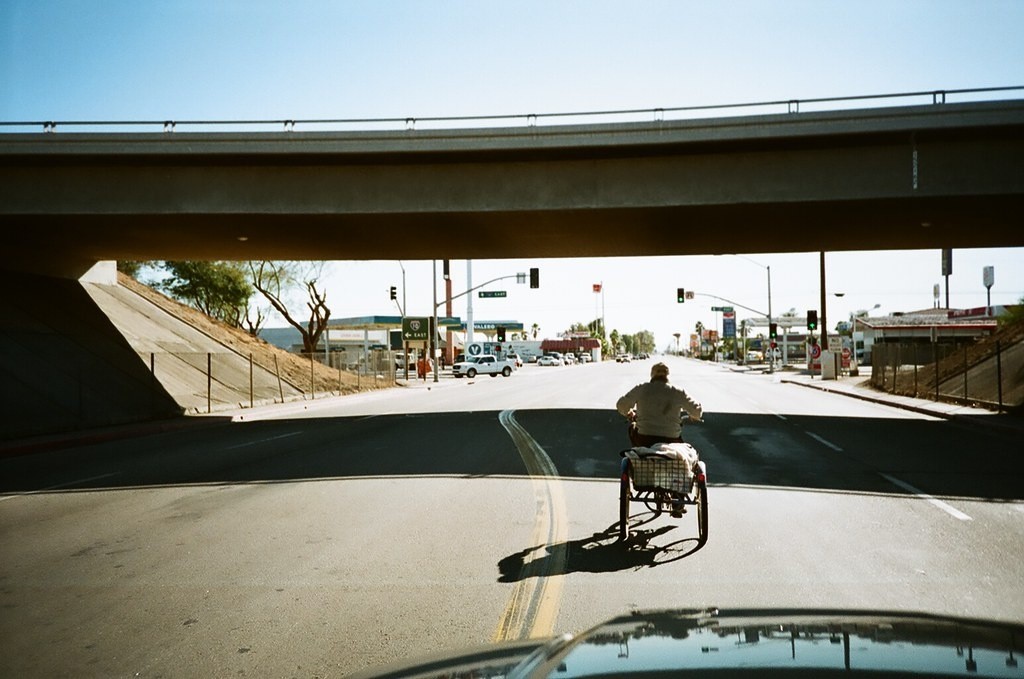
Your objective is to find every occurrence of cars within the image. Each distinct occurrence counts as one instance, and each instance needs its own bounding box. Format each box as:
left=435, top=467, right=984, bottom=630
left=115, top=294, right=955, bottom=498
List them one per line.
left=746, top=347, right=764, bottom=364
left=507, top=354, right=523, bottom=367
left=615, top=353, right=650, bottom=363
left=764, top=346, right=782, bottom=361
left=348, top=356, right=378, bottom=372
left=528, top=352, right=592, bottom=366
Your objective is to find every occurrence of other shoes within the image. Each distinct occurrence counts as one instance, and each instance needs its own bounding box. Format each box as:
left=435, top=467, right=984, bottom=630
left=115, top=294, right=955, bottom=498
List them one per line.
left=670, top=507, right=683, bottom=518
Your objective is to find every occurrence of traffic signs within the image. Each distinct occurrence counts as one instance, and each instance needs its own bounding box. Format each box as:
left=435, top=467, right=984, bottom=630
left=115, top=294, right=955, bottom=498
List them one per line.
left=401, top=316, right=430, bottom=340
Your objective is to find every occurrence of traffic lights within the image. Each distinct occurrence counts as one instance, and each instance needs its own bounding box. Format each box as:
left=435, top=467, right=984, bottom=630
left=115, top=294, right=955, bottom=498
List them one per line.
left=769, top=323, right=778, bottom=339
left=390, top=286, right=397, bottom=300
left=806, top=309, right=818, bottom=330
left=677, top=287, right=685, bottom=303
left=770, top=342, right=777, bottom=348
left=497, top=326, right=506, bottom=342
left=495, top=345, right=501, bottom=352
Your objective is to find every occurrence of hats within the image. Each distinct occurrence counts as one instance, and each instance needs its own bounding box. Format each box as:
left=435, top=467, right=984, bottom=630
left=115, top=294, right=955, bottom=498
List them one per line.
left=651, top=361, right=670, bottom=379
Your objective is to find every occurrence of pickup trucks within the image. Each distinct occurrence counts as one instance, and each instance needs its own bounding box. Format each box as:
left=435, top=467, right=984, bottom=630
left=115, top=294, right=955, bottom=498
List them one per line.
left=451, top=354, right=517, bottom=378
left=381, top=353, right=415, bottom=371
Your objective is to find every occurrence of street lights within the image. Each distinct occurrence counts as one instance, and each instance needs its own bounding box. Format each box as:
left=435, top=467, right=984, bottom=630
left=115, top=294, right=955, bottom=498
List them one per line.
left=834, top=292, right=858, bottom=367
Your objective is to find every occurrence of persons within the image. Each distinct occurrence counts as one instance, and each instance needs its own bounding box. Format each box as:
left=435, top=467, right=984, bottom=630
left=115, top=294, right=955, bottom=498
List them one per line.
left=577, top=352, right=582, bottom=362
left=441, top=354, right=446, bottom=370
left=617, top=362, right=702, bottom=518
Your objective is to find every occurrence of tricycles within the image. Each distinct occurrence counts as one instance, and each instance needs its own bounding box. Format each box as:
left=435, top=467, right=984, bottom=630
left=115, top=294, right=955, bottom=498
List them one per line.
left=617, top=407, right=709, bottom=544
